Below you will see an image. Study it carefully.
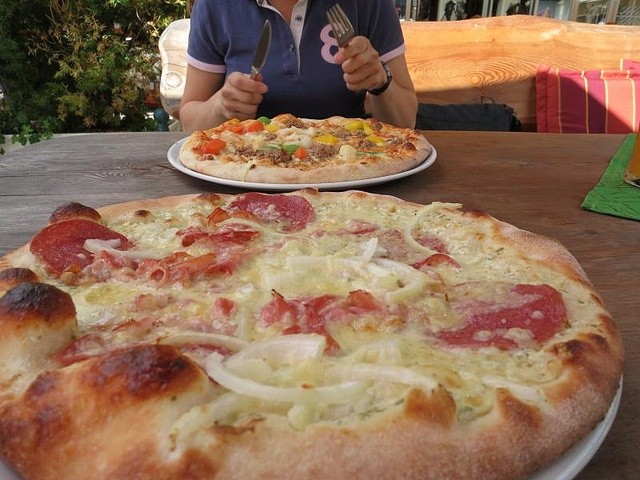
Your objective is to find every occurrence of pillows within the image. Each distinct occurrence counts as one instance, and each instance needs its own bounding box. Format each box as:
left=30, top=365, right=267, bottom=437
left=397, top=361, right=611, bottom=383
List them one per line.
left=537, top=59, right=639, bottom=134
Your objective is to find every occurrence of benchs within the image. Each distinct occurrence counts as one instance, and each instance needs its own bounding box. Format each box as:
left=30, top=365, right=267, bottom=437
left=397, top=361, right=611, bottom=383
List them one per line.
left=158, top=13, right=640, bottom=132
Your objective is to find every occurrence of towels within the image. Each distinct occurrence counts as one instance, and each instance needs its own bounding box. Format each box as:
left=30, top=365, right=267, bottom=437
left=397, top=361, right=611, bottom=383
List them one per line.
left=582, top=135, right=639, bottom=220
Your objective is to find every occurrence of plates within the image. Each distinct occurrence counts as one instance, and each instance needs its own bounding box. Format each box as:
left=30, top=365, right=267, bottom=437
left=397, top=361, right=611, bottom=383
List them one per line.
left=166, top=134, right=438, bottom=192
left=529, top=374, right=624, bottom=480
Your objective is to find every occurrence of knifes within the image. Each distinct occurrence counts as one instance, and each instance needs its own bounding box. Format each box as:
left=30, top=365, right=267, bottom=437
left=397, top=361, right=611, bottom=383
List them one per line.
left=250, top=19, right=272, bottom=80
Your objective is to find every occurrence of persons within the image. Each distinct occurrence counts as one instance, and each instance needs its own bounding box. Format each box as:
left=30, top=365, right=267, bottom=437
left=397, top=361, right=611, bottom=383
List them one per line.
left=179, top=0, right=419, bottom=130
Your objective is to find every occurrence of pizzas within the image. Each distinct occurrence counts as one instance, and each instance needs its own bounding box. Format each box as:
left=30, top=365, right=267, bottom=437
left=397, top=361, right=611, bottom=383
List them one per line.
left=177, top=112, right=432, bottom=185
left=0, top=185, right=625, bottom=479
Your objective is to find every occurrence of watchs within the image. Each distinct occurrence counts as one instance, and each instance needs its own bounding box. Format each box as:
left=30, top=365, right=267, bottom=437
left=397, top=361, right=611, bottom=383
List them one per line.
left=366, top=60, right=393, bottom=96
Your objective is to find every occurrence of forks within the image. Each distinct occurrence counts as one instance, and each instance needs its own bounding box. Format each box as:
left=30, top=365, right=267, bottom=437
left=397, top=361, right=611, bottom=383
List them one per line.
left=325, top=3, right=356, bottom=49
